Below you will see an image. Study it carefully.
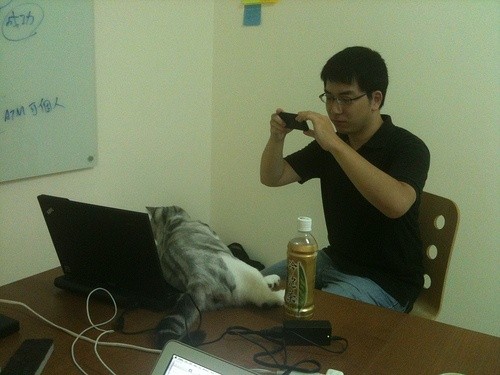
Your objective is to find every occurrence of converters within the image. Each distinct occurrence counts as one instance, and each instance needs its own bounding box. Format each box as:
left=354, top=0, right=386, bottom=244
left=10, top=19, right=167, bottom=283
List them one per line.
left=281, top=319, right=332, bottom=346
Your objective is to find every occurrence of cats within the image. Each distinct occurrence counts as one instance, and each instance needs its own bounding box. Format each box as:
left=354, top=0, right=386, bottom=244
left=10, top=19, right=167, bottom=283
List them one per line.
left=146, top=205, right=285, bottom=350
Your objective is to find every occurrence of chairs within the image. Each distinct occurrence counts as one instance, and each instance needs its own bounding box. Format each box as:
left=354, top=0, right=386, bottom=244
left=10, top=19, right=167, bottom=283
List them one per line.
left=399, top=189, right=460, bottom=320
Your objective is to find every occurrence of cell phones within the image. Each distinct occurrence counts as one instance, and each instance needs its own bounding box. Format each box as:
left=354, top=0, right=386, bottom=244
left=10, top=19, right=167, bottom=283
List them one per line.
left=279, top=112, right=309, bottom=131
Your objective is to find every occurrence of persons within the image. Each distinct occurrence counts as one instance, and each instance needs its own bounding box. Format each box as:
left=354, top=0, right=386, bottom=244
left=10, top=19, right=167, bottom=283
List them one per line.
left=258, top=46, right=430, bottom=314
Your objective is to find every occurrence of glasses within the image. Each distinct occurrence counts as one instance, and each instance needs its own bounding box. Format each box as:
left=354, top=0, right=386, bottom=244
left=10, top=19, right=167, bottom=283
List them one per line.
left=319, top=92, right=370, bottom=106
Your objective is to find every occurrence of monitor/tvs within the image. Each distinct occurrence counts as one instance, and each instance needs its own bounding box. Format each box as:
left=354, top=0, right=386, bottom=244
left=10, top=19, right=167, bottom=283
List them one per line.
left=149, top=339, right=260, bottom=375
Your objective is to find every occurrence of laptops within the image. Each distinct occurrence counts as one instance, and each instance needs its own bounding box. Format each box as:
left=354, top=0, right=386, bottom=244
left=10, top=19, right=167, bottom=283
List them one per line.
left=37, top=192, right=179, bottom=308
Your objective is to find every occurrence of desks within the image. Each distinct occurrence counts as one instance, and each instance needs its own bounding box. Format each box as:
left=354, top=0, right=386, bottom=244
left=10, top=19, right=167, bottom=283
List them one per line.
left=0, top=265, right=500, bottom=375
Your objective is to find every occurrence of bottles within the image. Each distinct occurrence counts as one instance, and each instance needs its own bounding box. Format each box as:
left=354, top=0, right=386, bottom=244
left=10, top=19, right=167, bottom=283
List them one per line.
left=282, top=216, right=318, bottom=320
